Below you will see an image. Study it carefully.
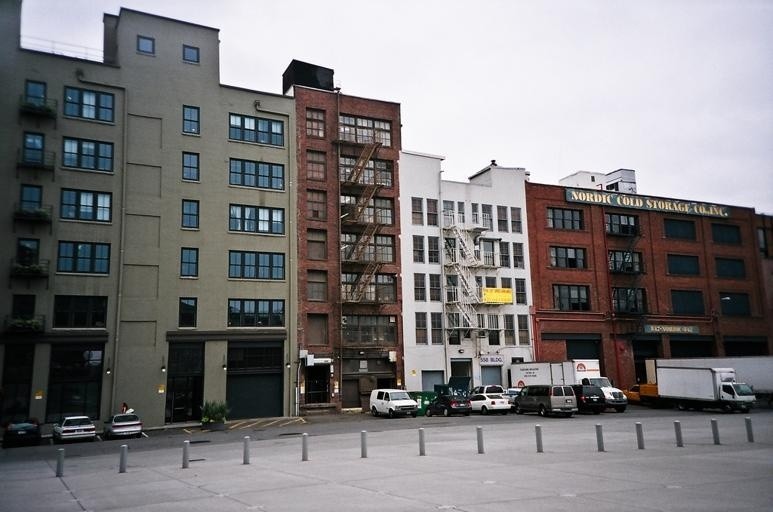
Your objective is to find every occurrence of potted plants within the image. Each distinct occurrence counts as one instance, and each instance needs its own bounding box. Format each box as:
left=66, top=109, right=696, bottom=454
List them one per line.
left=199, top=399, right=233, bottom=431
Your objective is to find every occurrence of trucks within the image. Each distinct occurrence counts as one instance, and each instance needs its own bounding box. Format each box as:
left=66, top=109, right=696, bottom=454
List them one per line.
left=510, top=359, right=628, bottom=412
left=623, top=384, right=658, bottom=404
left=655, top=366, right=757, bottom=414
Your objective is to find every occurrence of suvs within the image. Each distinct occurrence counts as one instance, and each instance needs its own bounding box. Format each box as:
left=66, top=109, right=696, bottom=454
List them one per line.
left=369, top=388, right=418, bottom=419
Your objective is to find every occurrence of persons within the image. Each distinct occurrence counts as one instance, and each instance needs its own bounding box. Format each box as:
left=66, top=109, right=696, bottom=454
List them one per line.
left=121, top=402, right=127, bottom=414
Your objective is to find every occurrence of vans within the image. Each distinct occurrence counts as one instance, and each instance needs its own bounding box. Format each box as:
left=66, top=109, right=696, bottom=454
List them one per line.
left=572, top=384, right=606, bottom=415
left=514, top=385, right=579, bottom=418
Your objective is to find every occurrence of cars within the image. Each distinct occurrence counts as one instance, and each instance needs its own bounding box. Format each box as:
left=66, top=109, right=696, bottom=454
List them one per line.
left=425, top=395, right=472, bottom=417
left=5, top=418, right=42, bottom=448
left=104, top=413, right=142, bottom=440
left=469, top=385, right=523, bottom=415
left=52, top=416, right=96, bottom=443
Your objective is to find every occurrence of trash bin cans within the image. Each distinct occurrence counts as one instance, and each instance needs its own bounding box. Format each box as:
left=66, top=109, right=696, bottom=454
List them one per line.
left=410, top=391, right=437, bottom=415
